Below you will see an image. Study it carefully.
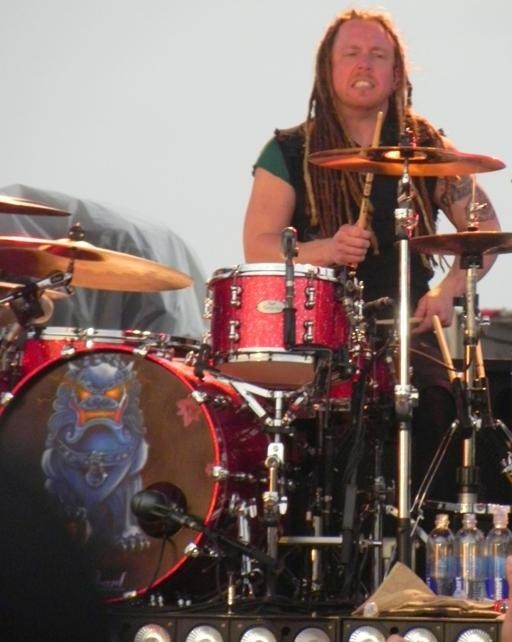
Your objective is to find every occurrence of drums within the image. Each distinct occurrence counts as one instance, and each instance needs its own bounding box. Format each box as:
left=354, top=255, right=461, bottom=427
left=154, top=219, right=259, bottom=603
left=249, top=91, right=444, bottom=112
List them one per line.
left=13, top=326, right=207, bottom=390
left=205, top=264, right=348, bottom=389
left=1, top=346, right=301, bottom=603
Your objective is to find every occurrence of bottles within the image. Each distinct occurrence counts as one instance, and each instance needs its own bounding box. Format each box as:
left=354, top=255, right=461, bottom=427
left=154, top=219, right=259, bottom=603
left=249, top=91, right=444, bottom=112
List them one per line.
left=423, top=512, right=512, bottom=602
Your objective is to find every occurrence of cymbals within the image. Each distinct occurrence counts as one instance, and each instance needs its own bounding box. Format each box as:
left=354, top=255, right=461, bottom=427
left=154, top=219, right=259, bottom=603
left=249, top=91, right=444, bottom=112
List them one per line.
left=1, top=233, right=192, bottom=292
left=1, top=197, right=68, bottom=217
left=409, top=233, right=511, bottom=255
left=307, top=147, right=506, bottom=177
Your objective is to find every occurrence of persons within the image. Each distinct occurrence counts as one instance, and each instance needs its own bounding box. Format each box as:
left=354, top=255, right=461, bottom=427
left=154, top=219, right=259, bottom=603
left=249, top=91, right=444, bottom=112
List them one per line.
left=244, top=10, right=501, bottom=484
